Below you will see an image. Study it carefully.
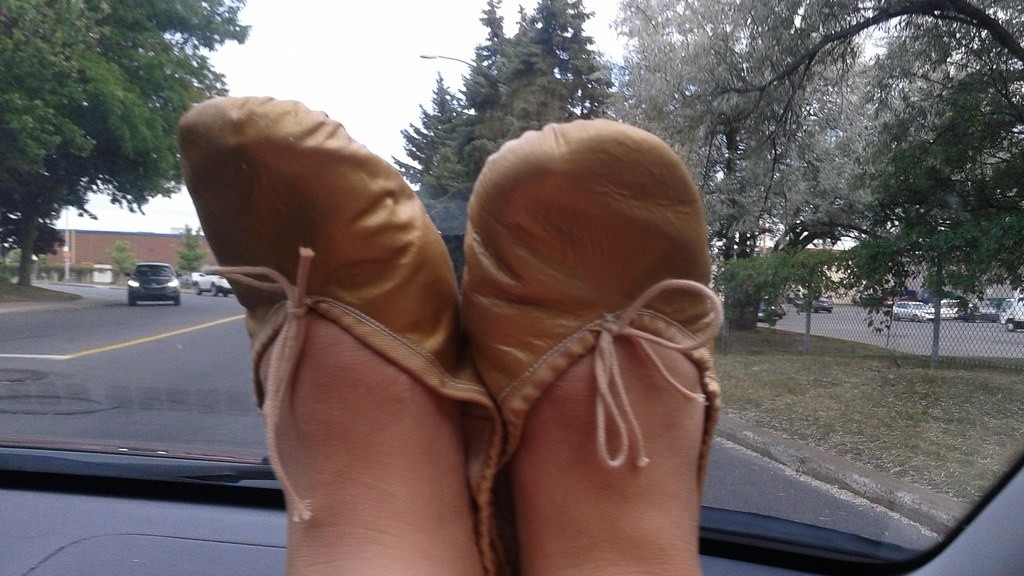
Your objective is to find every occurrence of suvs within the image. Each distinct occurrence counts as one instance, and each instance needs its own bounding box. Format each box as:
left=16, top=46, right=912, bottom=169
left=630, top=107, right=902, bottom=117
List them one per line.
left=999, top=299, right=1024, bottom=332
left=893, top=301, right=936, bottom=322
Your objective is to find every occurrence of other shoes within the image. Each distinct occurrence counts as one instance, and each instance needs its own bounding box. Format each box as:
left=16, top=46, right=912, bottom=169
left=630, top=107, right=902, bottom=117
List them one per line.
left=176, top=97, right=505, bottom=576
left=456, top=119, right=726, bottom=576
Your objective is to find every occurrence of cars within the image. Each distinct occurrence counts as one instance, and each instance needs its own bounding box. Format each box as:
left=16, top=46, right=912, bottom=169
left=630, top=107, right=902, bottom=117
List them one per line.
left=882, top=293, right=922, bottom=308
left=793, top=295, right=834, bottom=313
left=191, top=272, right=235, bottom=297
left=124, top=262, right=183, bottom=307
left=757, top=296, right=787, bottom=327
left=928, top=298, right=1000, bottom=323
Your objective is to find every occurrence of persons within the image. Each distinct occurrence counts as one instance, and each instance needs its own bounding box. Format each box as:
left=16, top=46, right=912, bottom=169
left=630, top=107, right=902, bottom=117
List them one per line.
left=180, top=97, right=722, bottom=576
